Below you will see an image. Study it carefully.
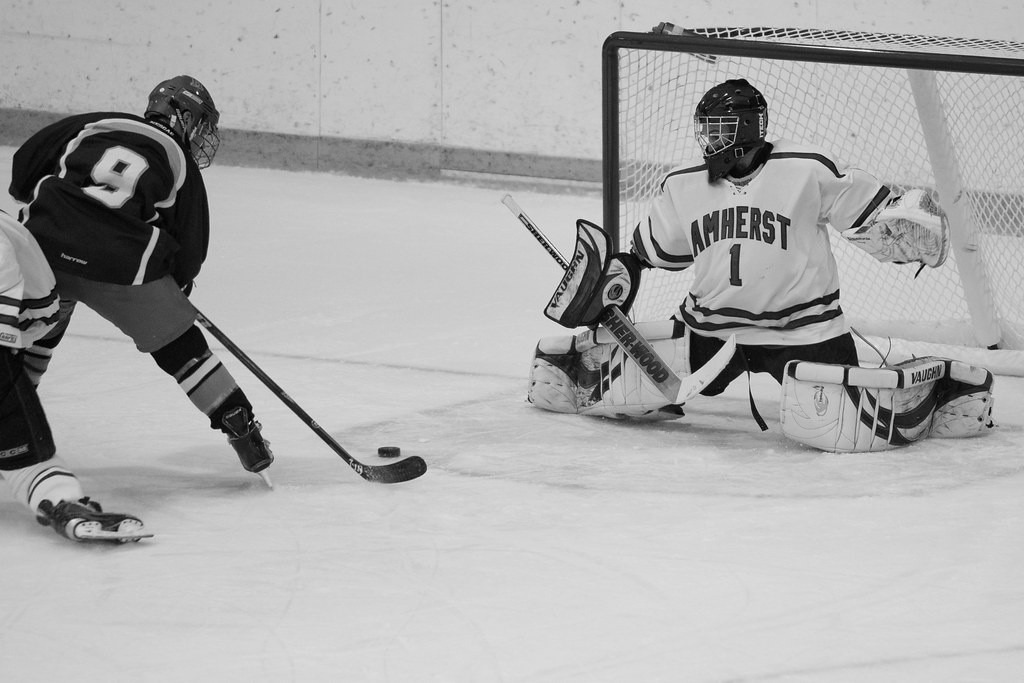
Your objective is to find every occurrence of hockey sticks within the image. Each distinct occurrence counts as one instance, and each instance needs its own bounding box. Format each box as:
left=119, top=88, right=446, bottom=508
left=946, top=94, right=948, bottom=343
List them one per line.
left=498, top=191, right=736, bottom=406
left=184, top=297, right=426, bottom=484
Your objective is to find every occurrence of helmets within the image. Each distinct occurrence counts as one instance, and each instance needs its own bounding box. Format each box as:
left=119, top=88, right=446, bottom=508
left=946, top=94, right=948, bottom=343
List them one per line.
left=144, top=75, right=220, bottom=145
left=693, top=78, right=769, bottom=183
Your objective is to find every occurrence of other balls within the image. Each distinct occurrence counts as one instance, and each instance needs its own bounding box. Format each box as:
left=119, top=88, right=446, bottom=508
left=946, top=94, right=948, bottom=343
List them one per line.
left=375, top=445, right=401, bottom=458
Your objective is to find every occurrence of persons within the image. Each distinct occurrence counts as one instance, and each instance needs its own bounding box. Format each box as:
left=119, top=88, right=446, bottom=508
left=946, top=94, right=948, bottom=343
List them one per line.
left=528, top=75, right=992, bottom=445
left=7, top=73, right=276, bottom=492
left=3, top=212, right=154, bottom=545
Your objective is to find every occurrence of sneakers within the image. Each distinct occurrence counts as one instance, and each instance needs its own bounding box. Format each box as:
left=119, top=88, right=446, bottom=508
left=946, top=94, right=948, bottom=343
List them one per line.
left=34, top=496, right=155, bottom=544
left=217, top=407, right=275, bottom=489
